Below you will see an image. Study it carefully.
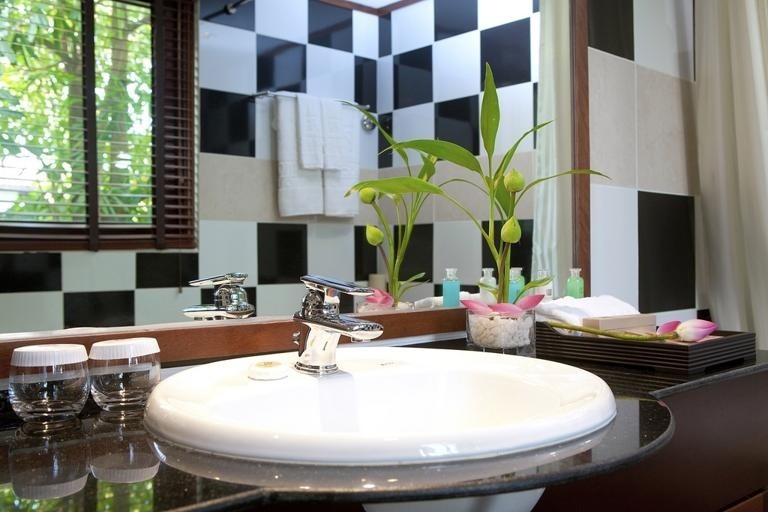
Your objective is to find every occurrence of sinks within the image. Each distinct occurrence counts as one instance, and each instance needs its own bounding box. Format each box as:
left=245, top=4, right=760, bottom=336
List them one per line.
left=142, top=347, right=616, bottom=465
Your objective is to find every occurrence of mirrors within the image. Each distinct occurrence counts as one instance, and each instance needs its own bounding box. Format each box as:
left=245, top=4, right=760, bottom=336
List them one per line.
left=0, top=0, right=591, bottom=379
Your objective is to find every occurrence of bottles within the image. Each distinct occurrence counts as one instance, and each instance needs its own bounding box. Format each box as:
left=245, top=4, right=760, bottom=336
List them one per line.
left=511, top=266, right=526, bottom=291
left=508, top=269, right=520, bottom=306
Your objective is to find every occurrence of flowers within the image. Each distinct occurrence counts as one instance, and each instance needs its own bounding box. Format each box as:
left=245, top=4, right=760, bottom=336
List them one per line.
left=378, top=60, right=614, bottom=327
left=349, top=96, right=439, bottom=316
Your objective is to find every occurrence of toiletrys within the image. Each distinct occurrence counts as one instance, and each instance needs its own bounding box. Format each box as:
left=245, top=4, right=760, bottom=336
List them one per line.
left=511, top=267, right=525, bottom=298
left=509, top=269, right=521, bottom=304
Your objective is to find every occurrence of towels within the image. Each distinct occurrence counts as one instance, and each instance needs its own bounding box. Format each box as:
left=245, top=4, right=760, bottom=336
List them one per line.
left=272, top=88, right=359, bottom=220
left=536, top=291, right=638, bottom=339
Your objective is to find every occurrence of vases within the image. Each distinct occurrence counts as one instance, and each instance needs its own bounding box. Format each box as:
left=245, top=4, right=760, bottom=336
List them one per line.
left=461, top=306, right=538, bottom=356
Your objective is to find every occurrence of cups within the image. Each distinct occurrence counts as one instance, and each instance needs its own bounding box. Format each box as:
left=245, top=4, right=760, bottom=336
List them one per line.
left=6, top=420, right=90, bottom=502
left=88, top=336, right=162, bottom=413
left=89, top=413, right=163, bottom=484
left=7, top=342, right=90, bottom=422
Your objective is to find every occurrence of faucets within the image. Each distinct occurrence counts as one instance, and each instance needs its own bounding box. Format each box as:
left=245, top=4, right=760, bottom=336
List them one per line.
left=292, top=274, right=383, bottom=378
left=180, top=273, right=254, bottom=321
